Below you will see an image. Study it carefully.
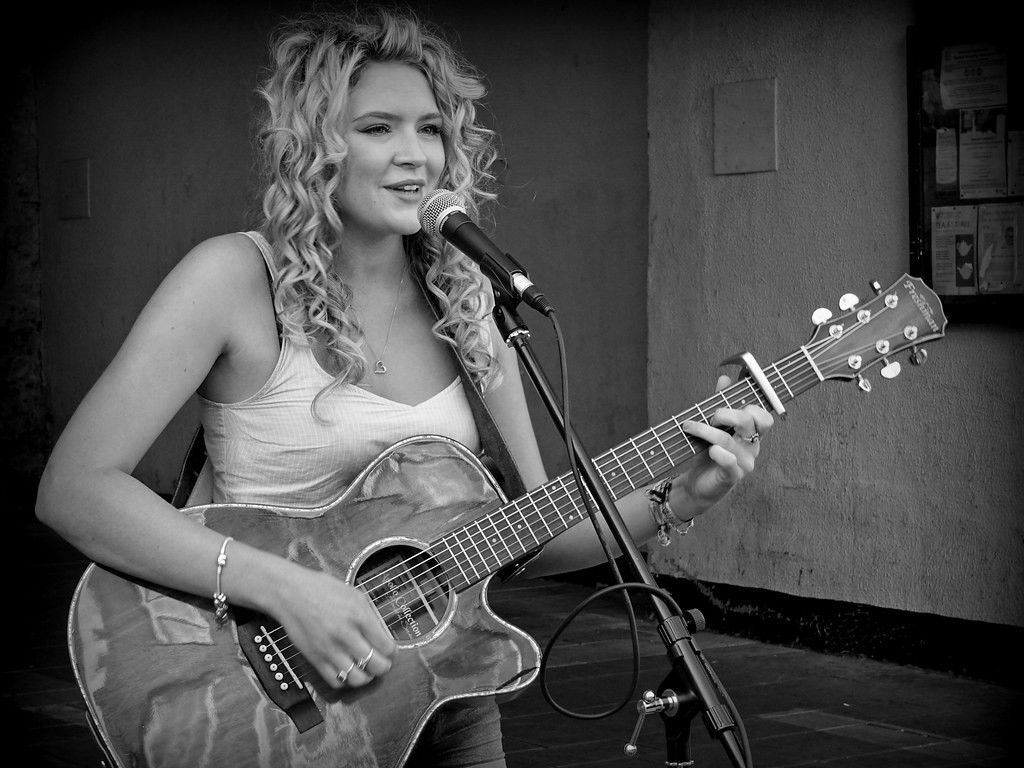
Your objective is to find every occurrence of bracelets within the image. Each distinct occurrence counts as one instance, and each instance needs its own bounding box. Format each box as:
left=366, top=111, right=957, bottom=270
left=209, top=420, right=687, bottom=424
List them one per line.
left=646, top=473, right=694, bottom=547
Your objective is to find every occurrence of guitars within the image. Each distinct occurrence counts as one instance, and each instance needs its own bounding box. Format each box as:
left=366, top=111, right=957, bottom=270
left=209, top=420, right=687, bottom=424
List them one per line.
left=65, top=272, right=948, bottom=768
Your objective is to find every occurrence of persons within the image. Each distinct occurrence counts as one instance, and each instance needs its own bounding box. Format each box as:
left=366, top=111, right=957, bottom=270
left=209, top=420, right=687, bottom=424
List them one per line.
left=35, top=9, right=773, bottom=768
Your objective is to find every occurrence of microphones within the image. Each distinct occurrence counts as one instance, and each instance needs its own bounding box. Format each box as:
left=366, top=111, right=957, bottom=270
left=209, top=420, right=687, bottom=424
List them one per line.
left=416, top=188, right=555, bottom=316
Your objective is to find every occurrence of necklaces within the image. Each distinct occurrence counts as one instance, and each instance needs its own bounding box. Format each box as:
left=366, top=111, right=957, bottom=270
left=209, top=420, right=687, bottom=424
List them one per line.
left=365, top=265, right=406, bottom=374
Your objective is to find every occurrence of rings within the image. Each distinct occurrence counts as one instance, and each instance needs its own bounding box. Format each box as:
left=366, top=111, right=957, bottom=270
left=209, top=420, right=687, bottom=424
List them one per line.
left=337, top=663, right=355, bottom=683
left=357, top=648, right=374, bottom=670
left=743, top=432, right=759, bottom=444
